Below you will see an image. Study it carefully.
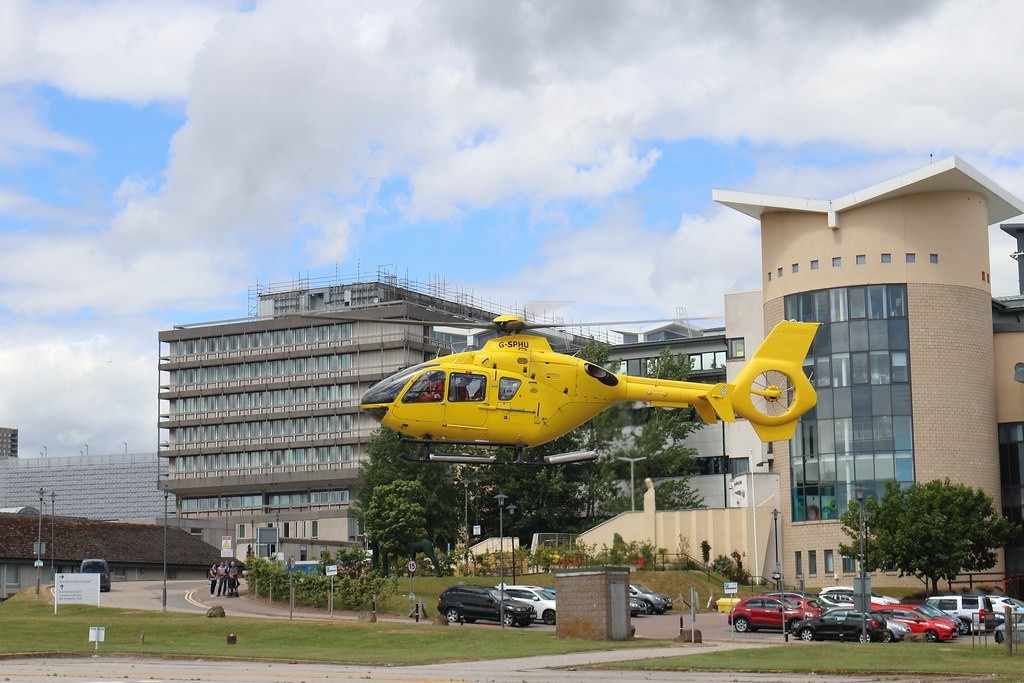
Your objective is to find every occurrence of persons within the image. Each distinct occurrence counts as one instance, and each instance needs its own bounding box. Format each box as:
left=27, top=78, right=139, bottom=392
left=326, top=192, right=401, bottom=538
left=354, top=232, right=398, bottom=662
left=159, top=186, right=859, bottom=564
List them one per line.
left=418, top=373, right=444, bottom=401
left=472, top=379, right=486, bottom=401
left=453, top=376, right=472, bottom=401
left=210, top=560, right=240, bottom=599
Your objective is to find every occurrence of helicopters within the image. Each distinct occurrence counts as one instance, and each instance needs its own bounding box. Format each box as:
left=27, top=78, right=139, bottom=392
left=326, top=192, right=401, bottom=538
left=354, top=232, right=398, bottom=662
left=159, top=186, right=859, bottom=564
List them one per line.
left=276, top=310, right=820, bottom=467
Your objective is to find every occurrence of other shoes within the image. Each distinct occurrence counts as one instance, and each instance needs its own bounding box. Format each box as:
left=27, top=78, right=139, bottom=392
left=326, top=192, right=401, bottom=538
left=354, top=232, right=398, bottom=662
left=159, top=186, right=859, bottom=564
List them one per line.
left=223, top=593, right=225, bottom=596
left=217, top=594, right=220, bottom=596
left=211, top=594, right=214, bottom=597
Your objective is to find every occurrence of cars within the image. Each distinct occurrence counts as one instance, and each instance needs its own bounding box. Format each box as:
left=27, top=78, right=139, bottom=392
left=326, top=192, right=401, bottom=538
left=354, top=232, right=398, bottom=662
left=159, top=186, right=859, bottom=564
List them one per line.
left=986, top=596, right=1024, bottom=624
left=766, top=585, right=964, bottom=644
left=630, top=598, right=648, bottom=617
left=522, top=587, right=556, bottom=600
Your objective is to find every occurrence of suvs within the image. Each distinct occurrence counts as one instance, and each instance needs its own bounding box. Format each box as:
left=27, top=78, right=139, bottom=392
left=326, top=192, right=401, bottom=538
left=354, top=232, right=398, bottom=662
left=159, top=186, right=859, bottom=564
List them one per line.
left=727, top=596, right=816, bottom=633
left=790, top=607, right=890, bottom=645
left=81, top=558, right=113, bottom=592
left=437, top=584, right=537, bottom=627
left=494, top=583, right=557, bottom=625
left=630, top=583, right=673, bottom=615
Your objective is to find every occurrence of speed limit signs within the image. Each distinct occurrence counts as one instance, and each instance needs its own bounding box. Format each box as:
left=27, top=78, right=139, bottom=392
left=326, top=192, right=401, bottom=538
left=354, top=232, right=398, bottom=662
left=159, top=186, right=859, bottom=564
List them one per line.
left=407, top=560, right=416, bottom=573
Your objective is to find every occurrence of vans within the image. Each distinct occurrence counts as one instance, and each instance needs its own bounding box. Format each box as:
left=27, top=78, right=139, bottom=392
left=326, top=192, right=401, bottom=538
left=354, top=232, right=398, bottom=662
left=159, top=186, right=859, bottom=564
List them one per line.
left=922, top=595, right=995, bottom=635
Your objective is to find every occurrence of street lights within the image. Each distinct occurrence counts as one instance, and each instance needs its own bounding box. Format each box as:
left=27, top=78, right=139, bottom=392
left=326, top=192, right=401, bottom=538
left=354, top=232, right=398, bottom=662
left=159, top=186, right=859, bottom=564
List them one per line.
left=616, top=455, right=647, bottom=511
left=34, top=486, right=46, bottom=599
left=460, top=478, right=473, bottom=577
left=224, top=496, right=231, bottom=536
left=770, top=507, right=782, bottom=591
left=162, top=491, right=173, bottom=611
left=504, top=502, right=518, bottom=585
left=47, top=491, right=58, bottom=585
left=852, top=483, right=870, bottom=644
left=493, top=492, right=510, bottom=629
